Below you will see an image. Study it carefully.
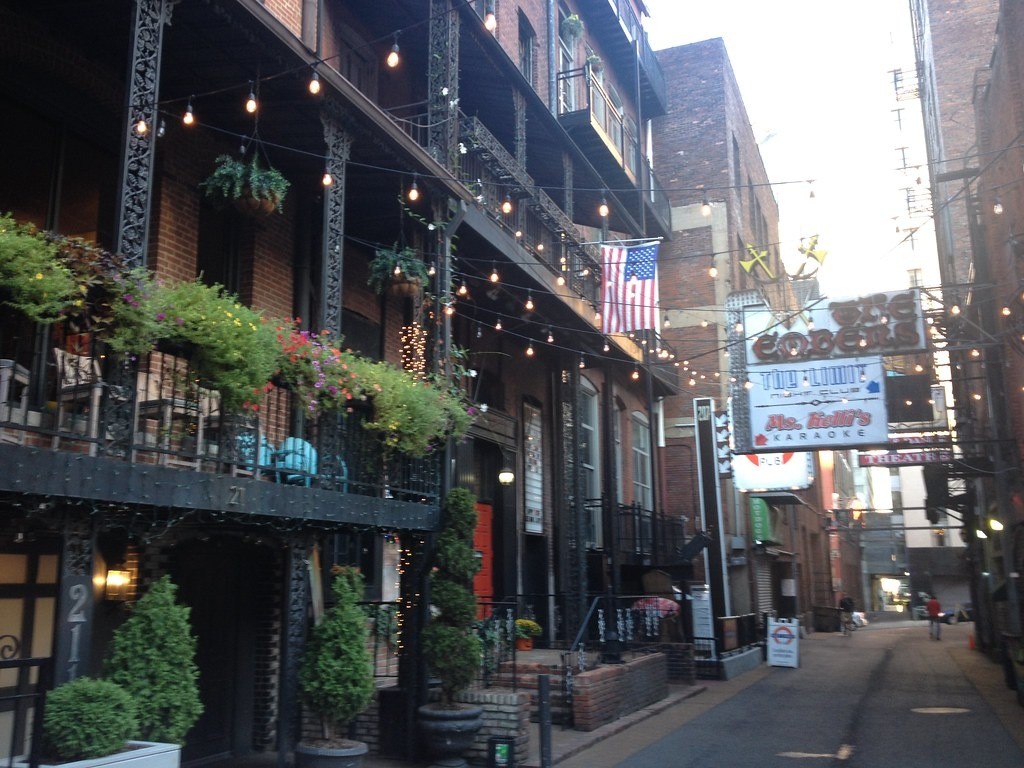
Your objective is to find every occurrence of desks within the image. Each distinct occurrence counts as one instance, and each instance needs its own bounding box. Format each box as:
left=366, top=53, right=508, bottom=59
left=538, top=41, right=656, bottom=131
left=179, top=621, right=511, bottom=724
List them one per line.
left=138, top=398, right=205, bottom=475
left=0, top=359, right=31, bottom=445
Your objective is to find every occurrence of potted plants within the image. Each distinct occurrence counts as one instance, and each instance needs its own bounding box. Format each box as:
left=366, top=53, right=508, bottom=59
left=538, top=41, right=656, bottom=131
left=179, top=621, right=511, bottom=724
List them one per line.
left=365, top=243, right=431, bottom=297
left=204, top=149, right=291, bottom=216
left=0, top=486, right=485, bottom=768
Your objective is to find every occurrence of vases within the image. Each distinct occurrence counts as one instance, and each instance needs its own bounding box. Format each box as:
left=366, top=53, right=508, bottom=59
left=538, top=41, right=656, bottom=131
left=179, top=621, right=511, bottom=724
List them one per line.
left=154, top=336, right=198, bottom=359
left=344, top=393, right=372, bottom=415
left=270, top=372, right=293, bottom=391
left=518, top=638, right=533, bottom=651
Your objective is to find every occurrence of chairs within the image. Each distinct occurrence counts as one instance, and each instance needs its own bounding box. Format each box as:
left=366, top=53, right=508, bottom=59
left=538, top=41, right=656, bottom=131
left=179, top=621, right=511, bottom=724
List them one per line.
left=53, top=346, right=350, bottom=494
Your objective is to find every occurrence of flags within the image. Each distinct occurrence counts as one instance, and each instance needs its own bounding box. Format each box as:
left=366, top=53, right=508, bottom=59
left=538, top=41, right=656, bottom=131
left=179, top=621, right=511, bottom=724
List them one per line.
left=601, top=243, right=660, bottom=333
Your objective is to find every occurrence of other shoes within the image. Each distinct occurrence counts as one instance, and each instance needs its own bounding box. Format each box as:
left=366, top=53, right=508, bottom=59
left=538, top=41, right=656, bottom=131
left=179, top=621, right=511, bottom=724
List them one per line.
left=930, top=632, right=933, bottom=638
left=937, top=637, right=940, bottom=640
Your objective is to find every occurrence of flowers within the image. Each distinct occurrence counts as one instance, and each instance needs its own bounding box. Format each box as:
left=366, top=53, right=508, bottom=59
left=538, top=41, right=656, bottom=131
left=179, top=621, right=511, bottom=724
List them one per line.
left=585, top=53, right=605, bottom=74
left=562, top=13, right=585, bottom=44
left=0, top=210, right=489, bottom=461
left=514, top=620, right=543, bottom=641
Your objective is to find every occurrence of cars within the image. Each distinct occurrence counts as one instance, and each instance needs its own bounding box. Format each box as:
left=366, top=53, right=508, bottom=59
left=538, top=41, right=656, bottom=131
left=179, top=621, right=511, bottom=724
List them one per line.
left=840, top=612, right=867, bottom=629
left=937, top=604, right=974, bottom=624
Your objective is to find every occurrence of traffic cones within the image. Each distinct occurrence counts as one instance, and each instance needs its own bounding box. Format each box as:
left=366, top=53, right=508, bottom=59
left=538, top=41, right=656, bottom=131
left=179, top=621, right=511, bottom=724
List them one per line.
left=970, top=636, right=976, bottom=650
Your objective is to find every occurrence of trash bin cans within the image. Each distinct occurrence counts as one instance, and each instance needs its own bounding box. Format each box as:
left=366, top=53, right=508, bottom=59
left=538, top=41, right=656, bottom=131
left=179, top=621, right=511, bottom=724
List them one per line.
left=811, top=605, right=846, bottom=632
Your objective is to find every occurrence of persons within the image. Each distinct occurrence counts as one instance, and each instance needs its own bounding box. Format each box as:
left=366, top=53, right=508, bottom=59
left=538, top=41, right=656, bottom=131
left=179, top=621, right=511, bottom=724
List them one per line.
left=840, top=592, right=855, bottom=631
left=927, top=596, right=941, bottom=640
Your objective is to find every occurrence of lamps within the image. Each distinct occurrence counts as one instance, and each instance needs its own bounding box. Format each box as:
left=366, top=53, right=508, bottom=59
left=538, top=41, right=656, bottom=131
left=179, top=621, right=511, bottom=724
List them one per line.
left=479, top=411, right=516, bottom=487
left=104, top=558, right=134, bottom=606
